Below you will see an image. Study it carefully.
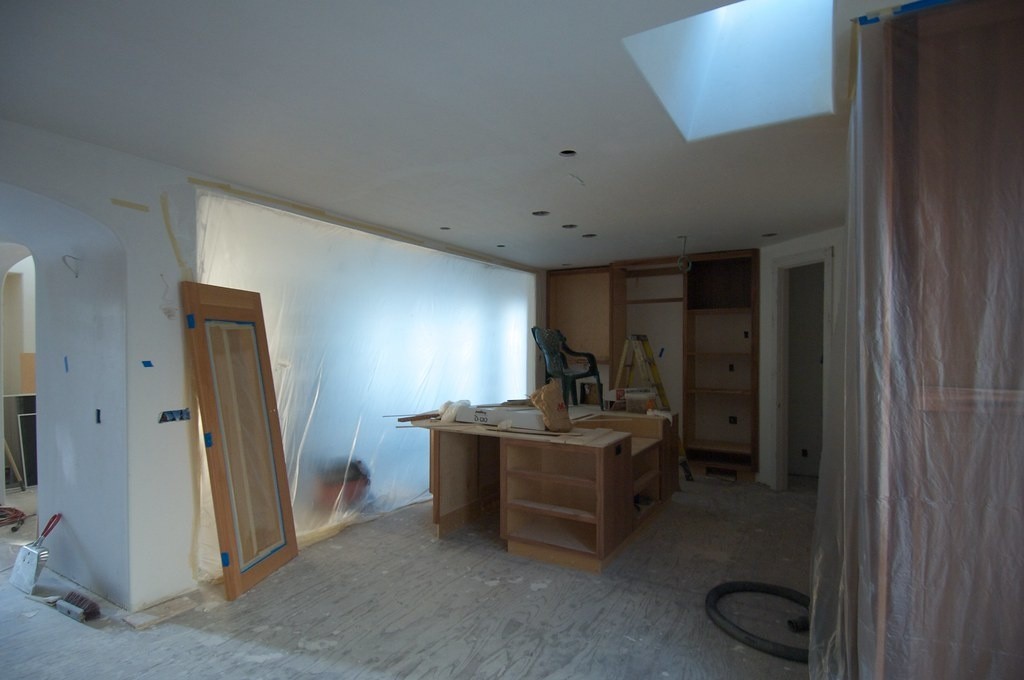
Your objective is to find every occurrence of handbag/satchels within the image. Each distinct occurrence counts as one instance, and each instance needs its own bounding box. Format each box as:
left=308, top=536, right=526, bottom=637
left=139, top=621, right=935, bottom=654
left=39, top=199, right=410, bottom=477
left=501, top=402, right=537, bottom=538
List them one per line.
left=528, top=377, right=572, bottom=433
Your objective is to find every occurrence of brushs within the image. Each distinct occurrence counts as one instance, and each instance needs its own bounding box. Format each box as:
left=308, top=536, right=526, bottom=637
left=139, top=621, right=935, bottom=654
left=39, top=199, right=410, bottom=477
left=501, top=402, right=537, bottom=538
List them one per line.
left=55, top=590, right=101, bottom=623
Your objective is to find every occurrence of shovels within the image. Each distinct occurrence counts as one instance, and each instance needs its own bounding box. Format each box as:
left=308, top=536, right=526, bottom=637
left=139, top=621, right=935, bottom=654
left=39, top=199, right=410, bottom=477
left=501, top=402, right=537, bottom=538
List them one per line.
left=9, top=512, right=62, bottom=595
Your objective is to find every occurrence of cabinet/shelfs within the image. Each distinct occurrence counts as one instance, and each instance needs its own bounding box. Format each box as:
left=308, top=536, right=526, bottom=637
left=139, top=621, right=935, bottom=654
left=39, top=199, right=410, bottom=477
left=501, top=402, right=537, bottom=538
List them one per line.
left=682, top=248, right=760, bottom=486
left=546, top=267, right=627, bottom=404
left=499, top=431, right=634, bottom=574
left=601, top=412, right=679, bottom=503
left=632, top=437, right=661, bottom=536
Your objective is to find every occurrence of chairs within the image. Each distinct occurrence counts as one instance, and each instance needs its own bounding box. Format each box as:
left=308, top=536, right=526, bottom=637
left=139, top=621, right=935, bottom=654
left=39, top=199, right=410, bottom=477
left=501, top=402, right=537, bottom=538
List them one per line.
left=532, top=326, right=604, bottom=414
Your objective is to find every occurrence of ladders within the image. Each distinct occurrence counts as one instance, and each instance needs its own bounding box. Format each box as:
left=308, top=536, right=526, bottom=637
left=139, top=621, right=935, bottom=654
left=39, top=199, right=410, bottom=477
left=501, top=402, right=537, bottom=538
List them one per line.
left=614, top=335, right=695, bottom=483
